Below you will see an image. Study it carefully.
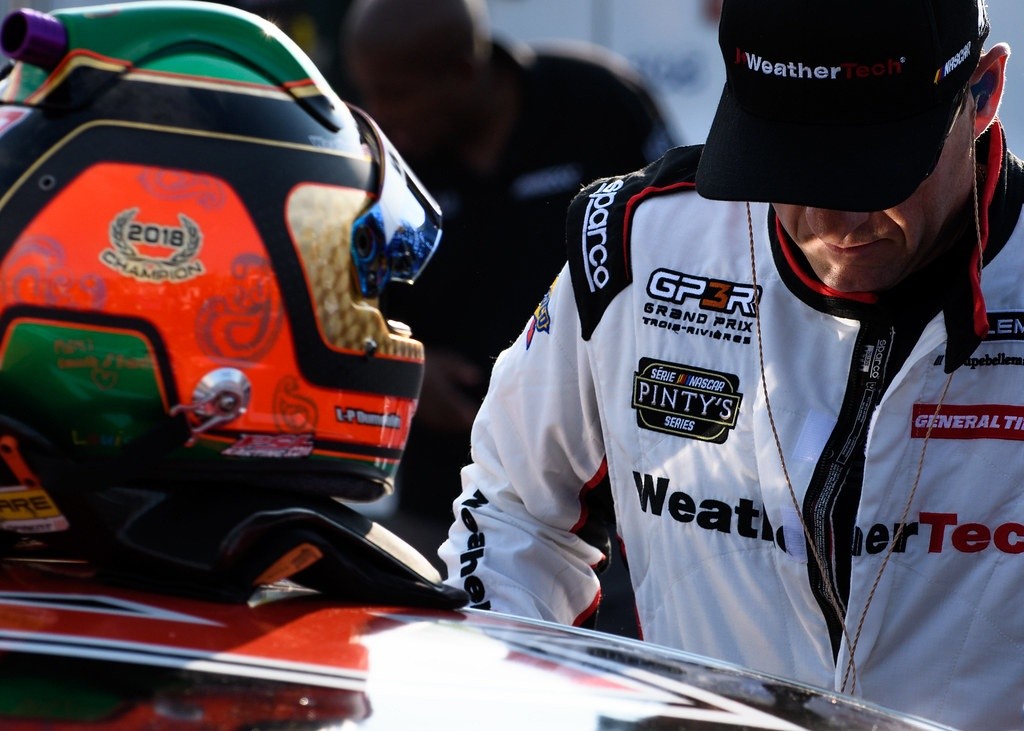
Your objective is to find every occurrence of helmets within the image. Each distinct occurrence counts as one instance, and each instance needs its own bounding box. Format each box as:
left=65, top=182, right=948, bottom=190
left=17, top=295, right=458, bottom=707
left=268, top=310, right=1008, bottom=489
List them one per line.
left=1, top=2, right=447, bottom=579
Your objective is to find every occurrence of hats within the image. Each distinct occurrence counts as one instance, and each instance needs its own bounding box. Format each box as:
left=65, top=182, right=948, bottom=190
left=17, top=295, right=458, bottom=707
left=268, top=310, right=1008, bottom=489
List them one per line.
left=697, top=0, right=989, bottom=214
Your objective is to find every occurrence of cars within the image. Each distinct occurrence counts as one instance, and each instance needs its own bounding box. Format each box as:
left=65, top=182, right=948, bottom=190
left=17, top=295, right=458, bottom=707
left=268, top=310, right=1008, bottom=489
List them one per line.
left=0, top=565, right=957, bottom=731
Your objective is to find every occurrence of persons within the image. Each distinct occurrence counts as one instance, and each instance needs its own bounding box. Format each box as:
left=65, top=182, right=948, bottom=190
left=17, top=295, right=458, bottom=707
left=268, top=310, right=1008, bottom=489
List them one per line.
left=437, top=1, right=1024, bottom=731
left=335, top=1, right=682, bottom=534
